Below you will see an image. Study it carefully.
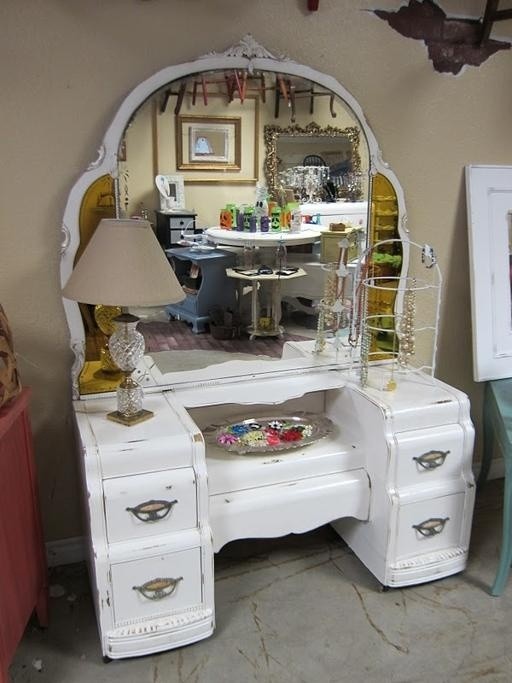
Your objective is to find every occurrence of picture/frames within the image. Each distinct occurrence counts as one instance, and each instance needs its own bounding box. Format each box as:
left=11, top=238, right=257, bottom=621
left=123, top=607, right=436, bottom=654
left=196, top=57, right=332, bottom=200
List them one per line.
left=463, top=164, right=512, bottom=385
left=154, top=90, right=259, bottom=186
left=175, top=112, right=242, bottom=172
left=157, top=174, right=185, bottom=209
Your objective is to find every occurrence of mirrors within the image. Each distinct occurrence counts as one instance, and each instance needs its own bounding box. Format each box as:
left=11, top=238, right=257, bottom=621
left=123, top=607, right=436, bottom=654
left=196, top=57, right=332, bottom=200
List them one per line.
left=60, top=37, right=410, bottom=408
left=265, top=122, right=363, bottom=203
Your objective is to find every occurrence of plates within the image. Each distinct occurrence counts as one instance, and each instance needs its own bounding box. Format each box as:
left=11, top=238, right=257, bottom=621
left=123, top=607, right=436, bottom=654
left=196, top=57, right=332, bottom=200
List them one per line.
left=203, top=411, right=335, bottom=454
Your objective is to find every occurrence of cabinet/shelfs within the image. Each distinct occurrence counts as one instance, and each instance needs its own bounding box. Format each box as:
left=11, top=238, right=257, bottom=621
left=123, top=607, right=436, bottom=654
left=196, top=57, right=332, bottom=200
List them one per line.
left=166, top=247, right=237, bottom=333
left=287, top=201, right=368, bottom=267
left=155, top=210, right=198, bottom=249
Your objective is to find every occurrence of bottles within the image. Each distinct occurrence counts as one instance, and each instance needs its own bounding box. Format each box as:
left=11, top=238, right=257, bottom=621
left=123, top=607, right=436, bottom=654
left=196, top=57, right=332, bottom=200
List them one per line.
left=276, top=240, right=287, bottom=272
left=219, top=194, right=302, bottom=233
left=254, top=181, right=269, bottom=229
left=307, top=211, right=322, bottom=225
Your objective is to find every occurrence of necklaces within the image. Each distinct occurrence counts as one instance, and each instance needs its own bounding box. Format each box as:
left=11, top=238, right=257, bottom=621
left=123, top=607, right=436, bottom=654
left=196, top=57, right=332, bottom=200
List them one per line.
left=357, top=278, right=372, bottom=388
left=396, top=283, right=418, bottom=371
left=313, top=263, right=364, bottom=354
left=347, top=246, right=374, bottom=350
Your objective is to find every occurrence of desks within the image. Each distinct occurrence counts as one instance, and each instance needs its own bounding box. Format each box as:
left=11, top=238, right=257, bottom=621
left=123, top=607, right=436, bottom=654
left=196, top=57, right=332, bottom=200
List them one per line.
left=204, top=224, right=324, bottom=344
left=479, top=378, right=511, bottom=597
left=1, top=385, right=52, bottom=683
left=73, top=362, right=482, bottom=663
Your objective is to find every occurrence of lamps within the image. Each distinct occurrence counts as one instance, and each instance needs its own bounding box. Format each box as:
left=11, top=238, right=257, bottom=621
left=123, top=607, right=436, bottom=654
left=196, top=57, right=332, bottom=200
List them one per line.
left=62, top=218, right=189, bottom=426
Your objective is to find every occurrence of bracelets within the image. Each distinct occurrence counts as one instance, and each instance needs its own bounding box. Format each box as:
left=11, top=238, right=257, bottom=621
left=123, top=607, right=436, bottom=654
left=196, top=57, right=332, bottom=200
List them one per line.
left=421, top=246, right=436, bottom=269
left=355, top=228, right=361, bottom=249
